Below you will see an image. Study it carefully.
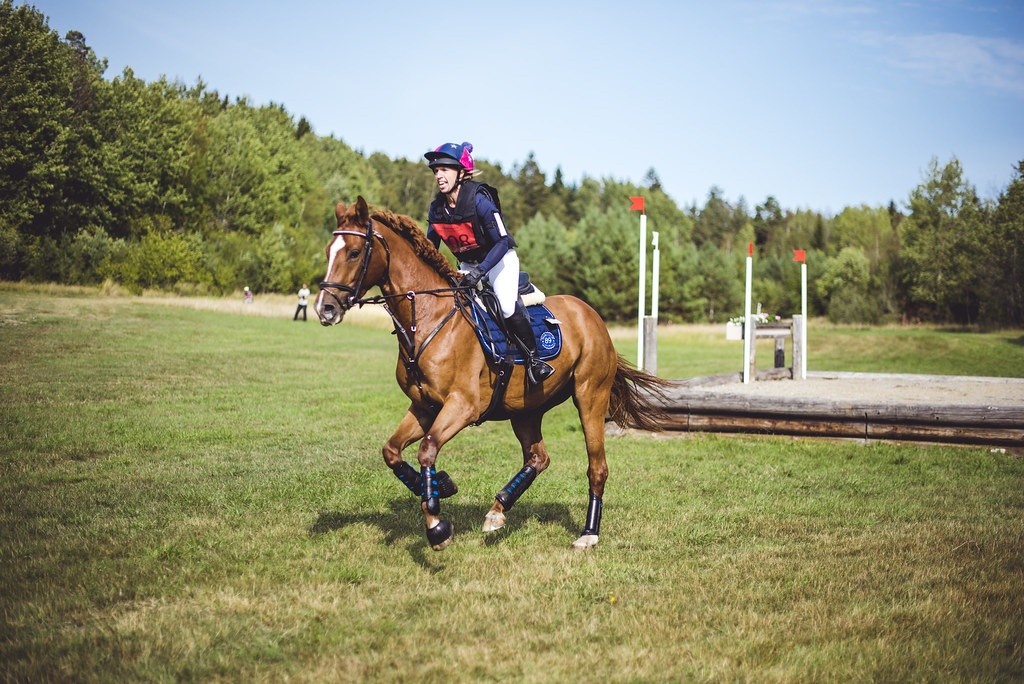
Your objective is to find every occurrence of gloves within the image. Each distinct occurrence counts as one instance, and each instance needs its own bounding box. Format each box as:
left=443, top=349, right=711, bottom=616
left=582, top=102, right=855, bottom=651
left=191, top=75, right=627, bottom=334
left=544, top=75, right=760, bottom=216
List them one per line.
left=462, top=265, right=485, bottom=288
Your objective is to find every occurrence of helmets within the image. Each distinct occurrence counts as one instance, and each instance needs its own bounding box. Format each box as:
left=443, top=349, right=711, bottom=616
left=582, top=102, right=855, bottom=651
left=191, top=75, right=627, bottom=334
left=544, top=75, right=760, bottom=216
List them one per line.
left=424, top=141, right=474, bottom=177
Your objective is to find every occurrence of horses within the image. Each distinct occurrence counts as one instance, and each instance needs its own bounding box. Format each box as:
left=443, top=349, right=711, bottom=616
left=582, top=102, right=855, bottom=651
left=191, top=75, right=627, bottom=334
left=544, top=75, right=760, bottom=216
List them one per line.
left=313, top=196, right=690, bottom=553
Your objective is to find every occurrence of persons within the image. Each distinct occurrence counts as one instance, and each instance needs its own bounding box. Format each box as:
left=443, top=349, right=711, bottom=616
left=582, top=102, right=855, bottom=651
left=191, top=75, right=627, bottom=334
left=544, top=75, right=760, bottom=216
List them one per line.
left=294, top=283, right=310, bottom=321
left=243, top=287, right=252, bottom=305
left=424, top=142, right=551, bottom=382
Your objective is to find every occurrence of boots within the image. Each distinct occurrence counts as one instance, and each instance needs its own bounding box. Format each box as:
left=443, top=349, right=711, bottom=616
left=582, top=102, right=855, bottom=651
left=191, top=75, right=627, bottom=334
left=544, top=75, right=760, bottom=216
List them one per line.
left=506, top=301, right=551, bottom=380
